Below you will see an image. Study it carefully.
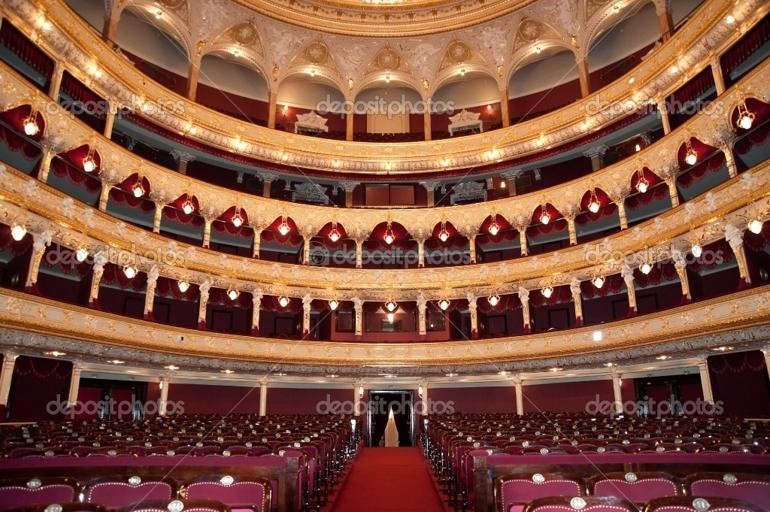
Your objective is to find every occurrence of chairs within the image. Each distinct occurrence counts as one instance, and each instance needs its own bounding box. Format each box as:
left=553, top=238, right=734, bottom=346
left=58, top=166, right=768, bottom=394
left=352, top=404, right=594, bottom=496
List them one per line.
left=0, top=415, right=361, bottom=511
left=419, top=409, right=769, bottom=512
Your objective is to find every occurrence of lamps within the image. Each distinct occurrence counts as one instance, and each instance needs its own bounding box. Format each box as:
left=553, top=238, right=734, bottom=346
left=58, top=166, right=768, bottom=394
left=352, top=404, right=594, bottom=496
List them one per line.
left=9, top=105, right=764, bottom=314
